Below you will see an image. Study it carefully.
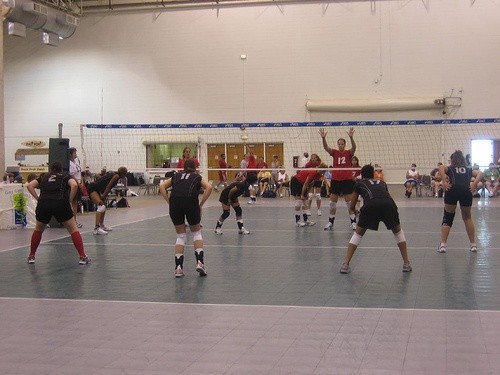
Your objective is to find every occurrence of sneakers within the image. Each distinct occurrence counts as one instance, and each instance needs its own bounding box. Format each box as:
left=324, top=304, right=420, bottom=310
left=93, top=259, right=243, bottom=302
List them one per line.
left=175, top=268, right=184, bottom=277
left=102, top=225, right=113, bottom=231
left=28, top=256, right=35, bottom=264
left=93, top=227, right=108, bottom=234
left=196, top=261, right=207, bottom=276
left=79, top=255, right=92, bottom=265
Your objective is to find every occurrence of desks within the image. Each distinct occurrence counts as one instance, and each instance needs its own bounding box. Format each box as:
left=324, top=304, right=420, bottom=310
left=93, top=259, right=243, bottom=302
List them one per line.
left=155, top=177, right=171, bottom=194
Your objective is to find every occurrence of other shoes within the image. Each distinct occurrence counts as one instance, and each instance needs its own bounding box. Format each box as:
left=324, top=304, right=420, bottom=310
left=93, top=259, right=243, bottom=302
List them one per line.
left=317, top=209, right=322, bottom=216
left=406, top=191, right=411, bottom=198
left=296, top=220, right=306, bottom=227
left=76, top=221, right=82, bottom=227
left=340, top=262, right=349, bottom=272
left=248, top=195, right=256, bottom=204
left=307, top=210, right=311, bottom=216
left=403, top=262, right=412, bottom=272
left=488, top=191, right=495, bottom=197
left=470, top=245, right=478, bottom=252
left=351, top=222, right=356, bottom=230
left=215, top=227, right=224, bottom=235
left=442, top=192, right=444, bottom=197
left=304, top=218, right=316, bottom=226
left=435, top=193, right=439, bottom=197
left=239, top=227, right=250, bottom=235
left=324, top=222, right=333, bottom=230
left=438, top=246, right=446, bottom=253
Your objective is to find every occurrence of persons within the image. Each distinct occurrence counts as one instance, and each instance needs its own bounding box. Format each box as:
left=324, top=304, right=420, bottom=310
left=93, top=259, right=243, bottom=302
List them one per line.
left=235, top=153, right=289, bottom=203
left=404, top=163, right=419, bottom=198
left=215, top=174, right=258, bottom=235
left=160, top=159, right=212, bottom=279
left=339, top=165, right=411, bottom=273
left=437, top=150, right=483, bottom=252
left=26, top=160, right=93, bottom=266
left=214, top=154, right=228, bottom=190
left=67, top=147, right=81, bottom=184
left=466, top=153, right=500, bottom=197
left=27, top=173, right=37, bottom=183
left=14, top=175, right=22, bottom=183
left=291, top=127, right=363, bottom=230
left=81, top=167, right=128, bottom=234
left=373, top=166, right=384, bottom=181
left=374, top=163, right=381, bottom=168
left=2, top=173, right=14, bottom=184
left=429, top=162, right=445, bottom=197
left=177, top=144, right=200, bottom=168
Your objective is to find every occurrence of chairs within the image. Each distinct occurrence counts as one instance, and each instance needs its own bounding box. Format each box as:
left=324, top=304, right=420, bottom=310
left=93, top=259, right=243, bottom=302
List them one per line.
left=138, top=176, right=160, bottom=195
left=113, top=177, right=127, bottom=197
left=275, top=186, right=291, bottom=197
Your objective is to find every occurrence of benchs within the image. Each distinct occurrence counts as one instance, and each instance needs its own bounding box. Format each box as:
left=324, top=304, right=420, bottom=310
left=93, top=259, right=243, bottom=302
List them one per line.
left=387, top=182, right=434, bottom=196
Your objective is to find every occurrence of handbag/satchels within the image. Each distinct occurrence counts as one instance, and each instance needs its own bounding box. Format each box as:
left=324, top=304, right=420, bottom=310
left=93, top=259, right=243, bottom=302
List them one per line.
left=262, top=190, right=276, bottom=198
left=117, top=198, right=129, bottom=207
